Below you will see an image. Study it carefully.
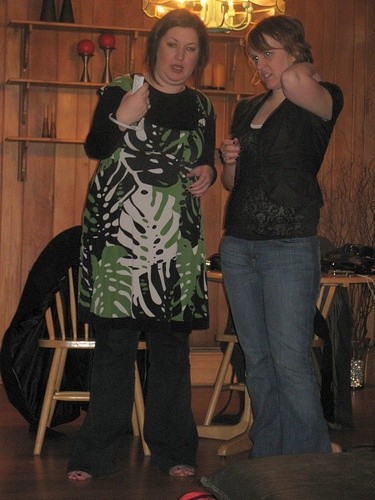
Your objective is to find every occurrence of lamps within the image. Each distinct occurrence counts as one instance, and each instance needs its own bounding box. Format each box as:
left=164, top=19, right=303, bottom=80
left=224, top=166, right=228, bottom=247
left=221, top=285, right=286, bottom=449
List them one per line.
left=142, top=0, right=286, bottom=33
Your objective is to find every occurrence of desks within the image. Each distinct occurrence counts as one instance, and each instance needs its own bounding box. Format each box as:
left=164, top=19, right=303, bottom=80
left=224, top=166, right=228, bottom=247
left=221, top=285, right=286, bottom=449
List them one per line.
left=195, top=268, right=375, bottom=456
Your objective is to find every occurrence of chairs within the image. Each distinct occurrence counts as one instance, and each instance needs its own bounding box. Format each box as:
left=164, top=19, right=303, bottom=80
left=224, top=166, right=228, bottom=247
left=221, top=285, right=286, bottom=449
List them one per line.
left=0, top=226, right=154, bottom=457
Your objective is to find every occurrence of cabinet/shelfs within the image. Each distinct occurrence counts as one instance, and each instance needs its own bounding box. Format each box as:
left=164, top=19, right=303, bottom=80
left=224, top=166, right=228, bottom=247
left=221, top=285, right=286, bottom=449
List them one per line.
left=4, top=20, right=258, bottom=149
